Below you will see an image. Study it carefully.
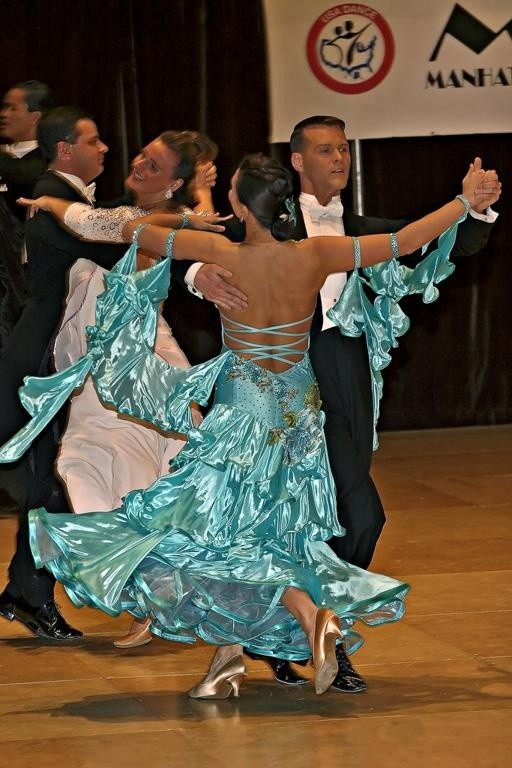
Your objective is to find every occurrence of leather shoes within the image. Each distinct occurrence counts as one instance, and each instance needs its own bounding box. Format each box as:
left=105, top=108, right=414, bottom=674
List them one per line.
left=0, top=584, right=83, bottom=641
left=244, top=646, right=309, bottom=685
left=331, top=642, right=368, bottom=692
left=113, top=616, right=153, bottom=648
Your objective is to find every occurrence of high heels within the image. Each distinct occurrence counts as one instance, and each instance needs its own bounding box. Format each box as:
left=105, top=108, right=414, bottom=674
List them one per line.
left=186, top=655, right=247, bottom=700
left=313, top=608, right=343, bottom=695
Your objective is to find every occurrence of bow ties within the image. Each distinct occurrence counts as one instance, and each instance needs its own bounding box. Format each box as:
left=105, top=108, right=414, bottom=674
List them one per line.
left=308, top=198, right=344, bottom=219
left=0, top=143, right=16, bottom=158
left=83, top=182, right=97, bottom=202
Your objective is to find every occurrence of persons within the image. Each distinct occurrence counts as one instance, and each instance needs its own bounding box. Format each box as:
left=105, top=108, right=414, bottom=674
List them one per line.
left=12, top=111, right=504, bottom=705
left=1, top=75, right=214, bottom=646
